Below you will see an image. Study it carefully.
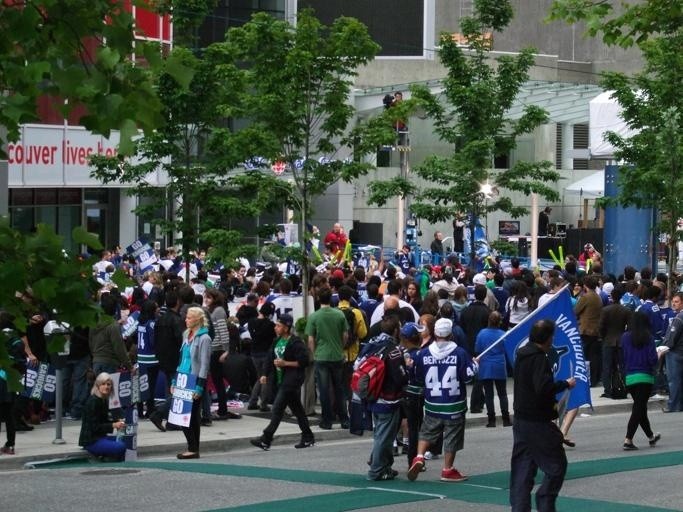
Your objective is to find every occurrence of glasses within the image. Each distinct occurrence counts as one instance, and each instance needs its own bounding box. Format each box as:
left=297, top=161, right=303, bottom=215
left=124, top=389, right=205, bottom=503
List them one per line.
left=408, top=288, right=417, bottom=291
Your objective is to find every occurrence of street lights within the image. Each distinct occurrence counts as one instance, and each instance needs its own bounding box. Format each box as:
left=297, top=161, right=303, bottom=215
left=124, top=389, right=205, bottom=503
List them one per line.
left=480, top=180, right=492, bottom=241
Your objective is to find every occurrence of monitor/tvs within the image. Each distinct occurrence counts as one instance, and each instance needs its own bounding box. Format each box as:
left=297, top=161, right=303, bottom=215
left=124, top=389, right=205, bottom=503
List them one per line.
left=499, top=221, right=520, bottom=236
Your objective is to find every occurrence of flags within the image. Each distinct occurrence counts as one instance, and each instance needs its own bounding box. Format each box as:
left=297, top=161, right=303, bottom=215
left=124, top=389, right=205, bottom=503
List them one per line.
left=503, top=289, right=592, bottom=411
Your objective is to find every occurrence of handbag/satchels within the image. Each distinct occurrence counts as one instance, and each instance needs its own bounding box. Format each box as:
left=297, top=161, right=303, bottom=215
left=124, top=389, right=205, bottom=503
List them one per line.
left=501, top=297, right=513, bottom=331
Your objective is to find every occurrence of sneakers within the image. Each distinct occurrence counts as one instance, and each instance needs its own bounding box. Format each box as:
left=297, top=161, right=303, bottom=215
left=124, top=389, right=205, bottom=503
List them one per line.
left=200, top=416, right=212, bottom=426
left=251, top=438, right=270, bottom=451
left=662, top=407, right=680, bottom=412
left=623, top=443, right=639, bottom=450
left=295, top=440, right=314, bottom=449
left=1, top=446, right=14, bottom=454
left=649, top=432, right=661, bottom=446
left=368, top=445, right=469, bottom=483
left=563, top=436, right=575, bottom=447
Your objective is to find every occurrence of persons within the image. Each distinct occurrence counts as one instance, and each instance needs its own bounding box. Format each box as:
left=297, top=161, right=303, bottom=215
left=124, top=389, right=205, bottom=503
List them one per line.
left=305, top=286, right=351, bottom=429
left=249, top=313, right=314, bottom=451
left=397, top=323, right=425, bottom=470
left=78, top=372, right=126, bottom=460
left=405, top=316, right=479, bottom=483
left=580, top=243, right=602, bottom=272
left=395, top=245, right=410, bottom=261
left=352, top=314, right=413, bottom=482
left=391, top=90, right=405, bottom=146
left=574, top=264, right=683, bottom=412
left=453, top=211, right=465, bottom=252
left=324, top=223, right=348, bottom=266
left=475, top=311, right=513, bottom=427
left=169, top=307, right=211, bottom=460
left=550, top=407, right=578, bottom=447
left=538, top=207, right=552, bottom=237
left=2, top=246, right=302, bottom=431
left=431, top=231, right=443, bottom=265
left=0, top=375, right=16, bottom=456
left=509, top=320, right=576, bottom=512
left=618, top=311, right=661, bottom=451
left=308, top=254, right=581, bottom=413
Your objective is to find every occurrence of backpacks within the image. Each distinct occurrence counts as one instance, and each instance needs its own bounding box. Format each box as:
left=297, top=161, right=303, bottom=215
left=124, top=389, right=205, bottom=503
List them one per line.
left=338, top=305, right=357, bottom=350
left=351, top=339, right=396, bottom=404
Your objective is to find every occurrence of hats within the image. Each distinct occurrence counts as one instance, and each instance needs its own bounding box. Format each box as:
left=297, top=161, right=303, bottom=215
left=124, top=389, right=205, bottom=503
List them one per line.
left=279, top=314, right=293, bottom=326
left=434, top=317, right=453, bottom=338
left=400, top=321, right=426, bottom=340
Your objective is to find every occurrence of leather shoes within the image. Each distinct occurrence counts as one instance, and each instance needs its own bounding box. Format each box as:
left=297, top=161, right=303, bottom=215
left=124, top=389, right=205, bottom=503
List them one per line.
left=248, top=405, right=260, bottom=410
left=177, top=454, right=200, bottom=459
left=17, top=424, right=34, bottom=431
left=150, top=416, right=166, bottom=432
left=223, top=411, right=242, bottom=420
left=503, top=422, right=513, bottom=427
left=486, top=423, right=496, bottom=427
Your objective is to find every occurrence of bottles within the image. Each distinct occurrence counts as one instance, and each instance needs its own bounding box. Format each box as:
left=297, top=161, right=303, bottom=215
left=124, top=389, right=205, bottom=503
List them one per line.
left=116, top=425, right=126, bottom=444
left=536, top=259, right=541, bottom=273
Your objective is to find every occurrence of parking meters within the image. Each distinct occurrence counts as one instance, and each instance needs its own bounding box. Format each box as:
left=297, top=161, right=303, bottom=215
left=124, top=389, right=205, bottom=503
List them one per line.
left=40, top=319, right=73, bottom=444
